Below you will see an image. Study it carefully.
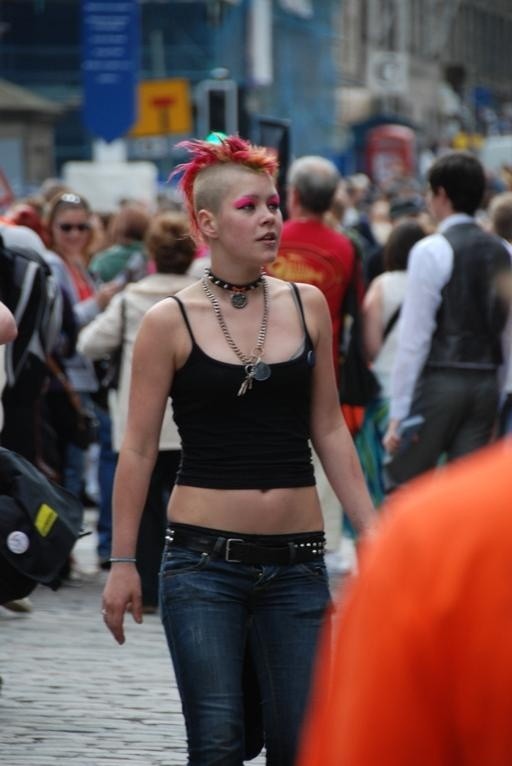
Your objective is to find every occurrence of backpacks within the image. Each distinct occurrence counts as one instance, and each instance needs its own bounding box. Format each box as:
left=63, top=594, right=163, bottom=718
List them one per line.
left=1, top=351, right=94, bottom=605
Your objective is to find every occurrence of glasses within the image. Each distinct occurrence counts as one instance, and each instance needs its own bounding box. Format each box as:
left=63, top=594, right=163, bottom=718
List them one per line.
left=59, top=223, right=89, bottom=231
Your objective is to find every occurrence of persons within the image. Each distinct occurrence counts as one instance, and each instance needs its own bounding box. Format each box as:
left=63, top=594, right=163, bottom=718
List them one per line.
left=295, top=435, right=512, bottom=766
left=0, top=172, right=212, bottom=613
left=262, top=154, right=512, bottom=574
left=102, top=131, right=380, bottom=766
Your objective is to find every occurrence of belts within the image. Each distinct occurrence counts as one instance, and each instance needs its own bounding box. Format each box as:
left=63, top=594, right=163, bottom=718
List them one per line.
left=165, top=528, right=325, bottom=565
